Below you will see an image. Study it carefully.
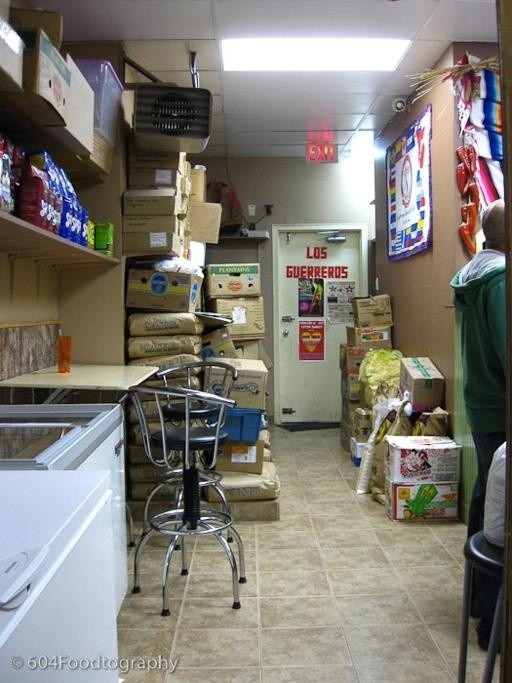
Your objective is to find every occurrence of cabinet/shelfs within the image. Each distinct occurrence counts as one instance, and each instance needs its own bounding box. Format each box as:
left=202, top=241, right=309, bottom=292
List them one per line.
left=2, top=88, right=122, bottom=278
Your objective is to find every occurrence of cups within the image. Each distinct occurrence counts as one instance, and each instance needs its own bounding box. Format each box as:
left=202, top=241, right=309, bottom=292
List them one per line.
left=56, top=335, right=72, bottom=374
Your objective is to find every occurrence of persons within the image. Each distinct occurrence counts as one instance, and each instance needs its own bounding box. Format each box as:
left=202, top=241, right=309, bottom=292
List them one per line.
left=476, top=443, right=507, bottom=652
left=449, top=199, right=507, bottom=617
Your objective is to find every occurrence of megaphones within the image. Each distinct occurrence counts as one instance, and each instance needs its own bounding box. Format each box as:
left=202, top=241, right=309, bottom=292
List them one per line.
left=392, top=98, right=407, bottom=111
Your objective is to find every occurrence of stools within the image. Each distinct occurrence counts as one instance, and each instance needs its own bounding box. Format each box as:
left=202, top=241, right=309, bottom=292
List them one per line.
left=457, top=532, right=512, bottom=683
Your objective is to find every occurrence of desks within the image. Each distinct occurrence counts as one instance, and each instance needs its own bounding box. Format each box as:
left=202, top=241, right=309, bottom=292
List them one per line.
left=1, top=360, right=158, bottom=404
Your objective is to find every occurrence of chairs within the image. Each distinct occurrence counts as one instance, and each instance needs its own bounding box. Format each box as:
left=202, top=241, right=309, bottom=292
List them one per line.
left=119, top=358, right=251, bottom=615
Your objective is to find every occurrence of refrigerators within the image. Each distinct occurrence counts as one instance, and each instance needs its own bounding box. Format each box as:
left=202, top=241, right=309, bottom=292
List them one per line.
left=0, top=400, right=130, bottom=623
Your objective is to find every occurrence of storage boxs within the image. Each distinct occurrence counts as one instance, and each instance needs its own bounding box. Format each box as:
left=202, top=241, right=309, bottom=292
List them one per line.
left=340, top=294, right=462, bottom=521
left=0, top=0, right=127, bottom=178
left=122, top=155, right=269, bottom=471
